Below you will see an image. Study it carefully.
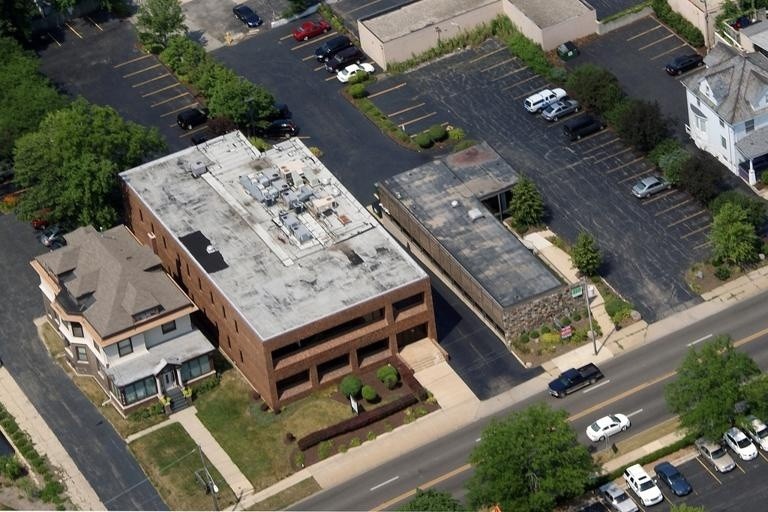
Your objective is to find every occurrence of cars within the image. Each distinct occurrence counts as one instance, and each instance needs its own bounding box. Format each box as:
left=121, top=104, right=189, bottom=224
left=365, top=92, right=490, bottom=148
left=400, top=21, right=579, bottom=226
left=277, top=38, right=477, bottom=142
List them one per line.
left=524, top=87, right=607, bottom=142
left=581, top=414, right=768, bottom=512
left=32, top=208, right=69, bottom=253
left=632, top=176, right=673, bottom=199
left=293, top=19, right=375, bottom=84
left=177, top=103, right=300, bottom=146
left=233, top=4, right=263, bottom=28
left=665, top=54, right=704, bottom=76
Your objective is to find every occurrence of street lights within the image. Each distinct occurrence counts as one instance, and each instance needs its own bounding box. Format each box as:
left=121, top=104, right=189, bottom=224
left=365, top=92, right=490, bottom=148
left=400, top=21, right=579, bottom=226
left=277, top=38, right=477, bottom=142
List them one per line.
left=194, top=446, right=219, bottom=511
left=571, top=276, right=597, bottom=356
left=244, top=97, right=256, bottom=142
left=700, top=0, right=711, bottom=53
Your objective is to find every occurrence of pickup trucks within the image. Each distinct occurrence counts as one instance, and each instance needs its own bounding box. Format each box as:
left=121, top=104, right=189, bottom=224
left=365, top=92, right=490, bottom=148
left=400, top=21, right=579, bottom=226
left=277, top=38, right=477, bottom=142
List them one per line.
left=548, top=362, right=604, bottom=399
